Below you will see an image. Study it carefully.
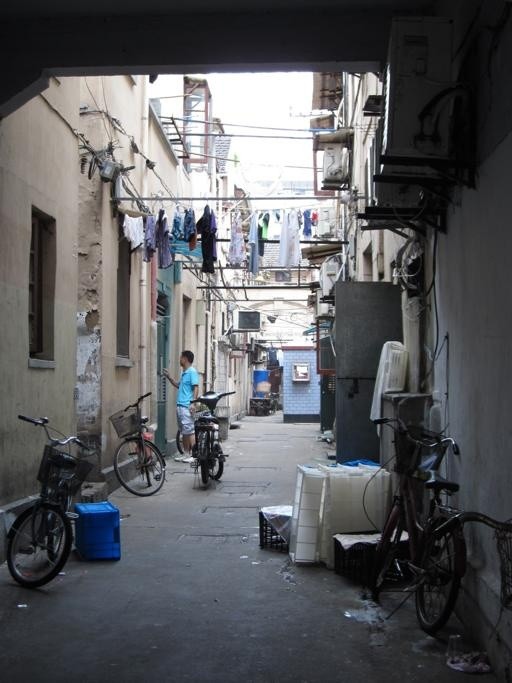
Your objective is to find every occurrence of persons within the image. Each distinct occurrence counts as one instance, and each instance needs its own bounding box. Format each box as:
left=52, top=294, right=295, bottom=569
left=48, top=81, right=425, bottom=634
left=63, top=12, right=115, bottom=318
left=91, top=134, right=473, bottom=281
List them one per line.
left=161, top=349, right=200, bottom=463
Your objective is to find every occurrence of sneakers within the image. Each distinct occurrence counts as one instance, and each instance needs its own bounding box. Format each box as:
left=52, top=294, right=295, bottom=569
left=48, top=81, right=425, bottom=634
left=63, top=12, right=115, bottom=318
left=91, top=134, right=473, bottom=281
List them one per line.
left=175, top=453, right=196, bottom=463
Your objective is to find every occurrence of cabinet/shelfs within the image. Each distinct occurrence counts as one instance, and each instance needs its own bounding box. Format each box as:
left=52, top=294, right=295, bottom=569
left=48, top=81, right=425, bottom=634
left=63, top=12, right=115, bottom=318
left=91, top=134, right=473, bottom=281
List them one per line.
left=379, top=394, right=432, bottom=473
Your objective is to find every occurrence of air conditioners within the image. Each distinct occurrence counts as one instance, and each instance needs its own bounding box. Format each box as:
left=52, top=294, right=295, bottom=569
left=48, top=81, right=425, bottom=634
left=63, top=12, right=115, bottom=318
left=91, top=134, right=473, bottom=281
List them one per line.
left=316, top=288, right=329, bottom=315
left=323, top=143, right=349, bottom=186
left=320, top=262, right=338, bottom=298
left=316, top=207, right=337, bottom=236
left=244, top=332, right=252, bottom=344
left=364, top=145, right=419, bottom=227
left=380, top=15, right=456, bottom=179
left=233, top=310, right=262, bottom=332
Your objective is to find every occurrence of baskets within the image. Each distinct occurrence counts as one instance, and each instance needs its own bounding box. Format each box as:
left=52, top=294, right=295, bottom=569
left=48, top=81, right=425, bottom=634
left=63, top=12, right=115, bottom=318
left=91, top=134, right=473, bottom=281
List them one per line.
left=331, top=527, right=383, bottom=576
left=258, top=506, right=293, bottom=550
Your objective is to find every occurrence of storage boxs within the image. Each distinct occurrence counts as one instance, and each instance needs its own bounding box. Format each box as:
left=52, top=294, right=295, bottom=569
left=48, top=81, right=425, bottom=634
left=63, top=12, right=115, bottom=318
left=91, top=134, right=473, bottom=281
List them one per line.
left=288, top=463, right=392, bottom=564
left=74, top=501, right=121, bottom=560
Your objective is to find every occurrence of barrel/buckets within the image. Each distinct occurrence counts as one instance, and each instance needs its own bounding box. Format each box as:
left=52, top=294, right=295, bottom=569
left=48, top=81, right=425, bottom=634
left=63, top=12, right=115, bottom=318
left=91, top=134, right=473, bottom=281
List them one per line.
left=253, top=370, right=269, bottom=399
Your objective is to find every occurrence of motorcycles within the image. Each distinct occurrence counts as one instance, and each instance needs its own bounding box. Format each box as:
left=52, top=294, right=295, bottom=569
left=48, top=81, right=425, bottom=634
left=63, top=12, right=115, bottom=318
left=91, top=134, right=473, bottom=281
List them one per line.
left=190, top=390, right=235, bottom=489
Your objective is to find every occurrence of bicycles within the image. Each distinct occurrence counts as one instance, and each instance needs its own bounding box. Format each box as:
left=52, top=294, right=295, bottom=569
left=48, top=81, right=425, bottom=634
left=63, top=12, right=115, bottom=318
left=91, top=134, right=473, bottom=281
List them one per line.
left=434, top=510, right=512, bottom=614
left=369, top=416, right=466, bottom=633
left=6, top=414, right=94, bottom=587
left=108, top=391, right=166, bottom=496
left=176, top=429, right=184, bottom=454
